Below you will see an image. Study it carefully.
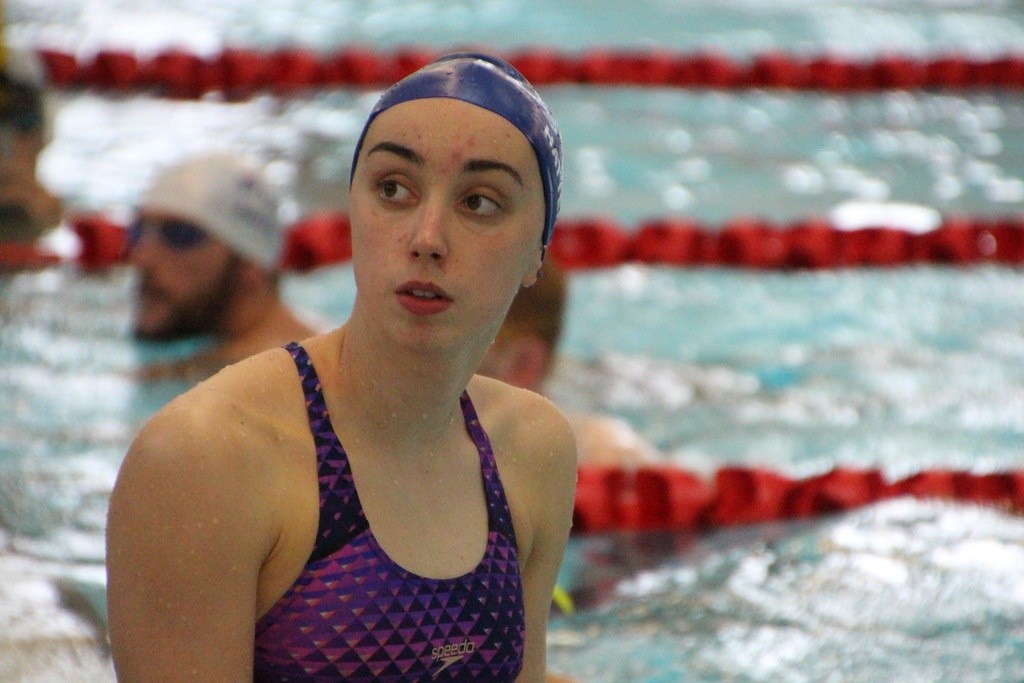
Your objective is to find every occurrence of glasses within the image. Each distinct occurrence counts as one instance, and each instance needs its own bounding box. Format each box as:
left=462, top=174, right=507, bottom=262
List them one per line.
left=128, top=215, right=204, bottom=247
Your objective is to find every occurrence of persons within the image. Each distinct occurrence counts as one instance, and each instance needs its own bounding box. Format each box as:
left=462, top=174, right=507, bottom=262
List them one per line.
left=0, top=44, right=77, bottom=248
left=107, top=52, right=581, bottom=680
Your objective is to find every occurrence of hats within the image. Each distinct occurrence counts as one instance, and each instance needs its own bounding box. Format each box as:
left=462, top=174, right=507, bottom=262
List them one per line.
left=133, top=152, right=283, bottom=273
left=350, top=57, right=562, bottom=260
